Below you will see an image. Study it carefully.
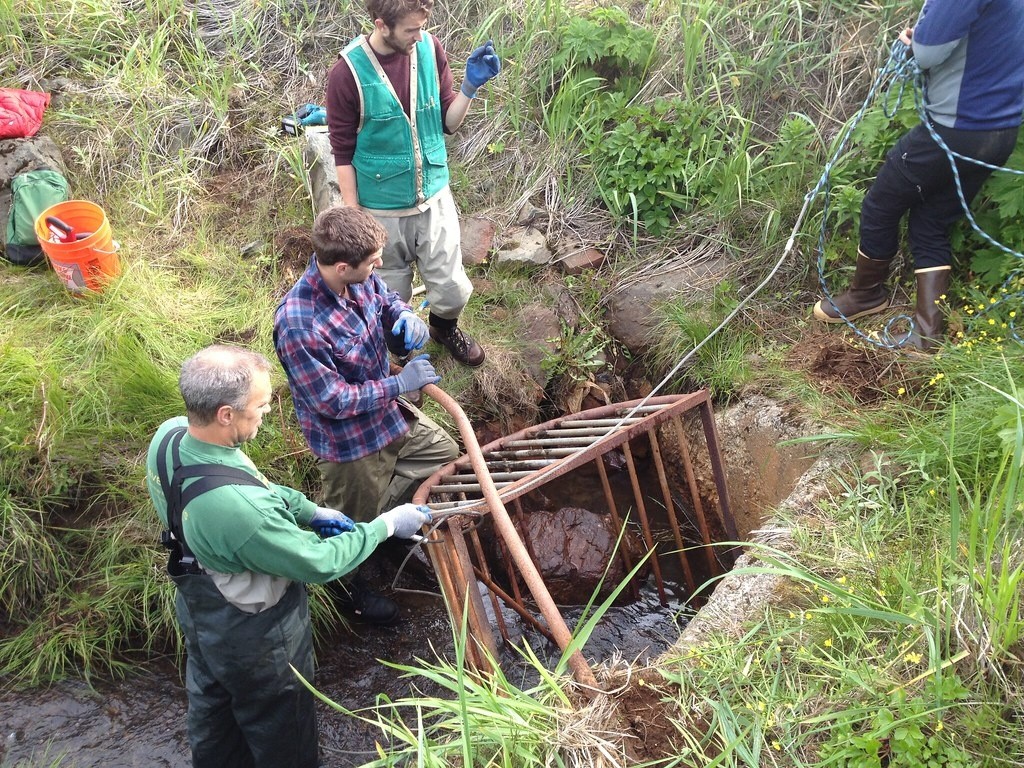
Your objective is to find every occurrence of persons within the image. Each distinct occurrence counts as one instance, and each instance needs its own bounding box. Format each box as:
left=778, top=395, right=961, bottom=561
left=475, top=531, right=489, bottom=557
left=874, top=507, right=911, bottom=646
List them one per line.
left=272, top=205, right=462, bottom=625
left=814, top=0, right=1023, bottom=353
left=326, top=0, right=504, bottom=363
left=143, top=340, right=432, bottom=767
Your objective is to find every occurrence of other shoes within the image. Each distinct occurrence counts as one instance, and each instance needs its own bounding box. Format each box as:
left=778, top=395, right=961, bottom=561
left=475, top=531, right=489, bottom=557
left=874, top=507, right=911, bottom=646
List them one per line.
left=328, top=572, right=399, bottom=624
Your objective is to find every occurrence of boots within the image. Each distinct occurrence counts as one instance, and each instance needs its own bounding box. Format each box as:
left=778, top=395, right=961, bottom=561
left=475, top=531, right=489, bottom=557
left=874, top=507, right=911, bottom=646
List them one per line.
left=872, top=265, right=952, bottom=354
left=428, top=311, right=486, bottom=367
left=812, top=244, right=900, bottom=322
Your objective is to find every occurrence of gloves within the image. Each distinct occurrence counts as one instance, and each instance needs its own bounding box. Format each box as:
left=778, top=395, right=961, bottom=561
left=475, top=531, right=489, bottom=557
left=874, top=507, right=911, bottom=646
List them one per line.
left=391, top=311, right=430, bottom=350
left=377, top=502, right=433, bottom=544
left=394, top=353, right=442, bottom=395
left=460, top=40, right=501, bottom=98
left=308, top=505, right=355, bottom=538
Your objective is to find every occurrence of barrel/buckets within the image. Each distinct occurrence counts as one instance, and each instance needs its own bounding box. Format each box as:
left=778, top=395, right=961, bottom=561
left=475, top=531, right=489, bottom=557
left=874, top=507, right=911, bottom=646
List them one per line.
left=35, top=200, right=121, bottom=297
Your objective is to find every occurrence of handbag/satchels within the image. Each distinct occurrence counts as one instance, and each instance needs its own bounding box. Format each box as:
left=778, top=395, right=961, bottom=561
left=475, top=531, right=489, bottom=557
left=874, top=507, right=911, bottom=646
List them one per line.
left=0, top=86, right=50, bottom=139
left=5, top=170, right=69, bottom=267
